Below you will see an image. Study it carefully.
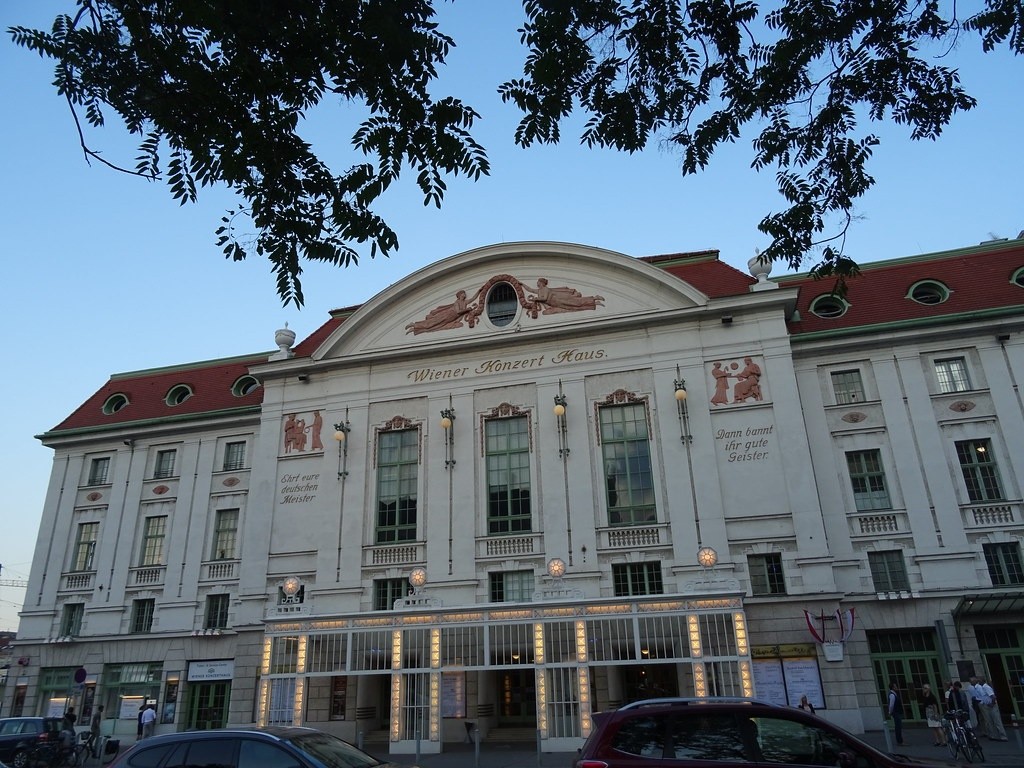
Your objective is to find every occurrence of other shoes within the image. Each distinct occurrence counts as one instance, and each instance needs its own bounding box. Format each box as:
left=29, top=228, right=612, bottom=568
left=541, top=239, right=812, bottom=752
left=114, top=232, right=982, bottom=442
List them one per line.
left=939, top=743, right=947, bottom=747
left=897, top=743, right=910, bottom=746
left=982, top=734, right=1008, bottom=742
left=934, top=742, right=940, bottom=746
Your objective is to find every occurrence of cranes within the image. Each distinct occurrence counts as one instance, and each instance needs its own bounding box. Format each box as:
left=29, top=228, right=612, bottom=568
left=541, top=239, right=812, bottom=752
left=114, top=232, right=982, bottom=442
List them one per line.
left=1, top=564, right=29, bottom=587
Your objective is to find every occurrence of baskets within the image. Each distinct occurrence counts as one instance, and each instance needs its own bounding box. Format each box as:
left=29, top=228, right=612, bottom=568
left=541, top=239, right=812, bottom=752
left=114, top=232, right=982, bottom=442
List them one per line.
left=80, top=731, right=89, bottom=740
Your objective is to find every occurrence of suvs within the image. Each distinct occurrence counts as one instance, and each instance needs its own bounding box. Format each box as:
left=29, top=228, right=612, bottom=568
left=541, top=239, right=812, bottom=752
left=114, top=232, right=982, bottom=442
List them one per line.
left=574, top=699, right=958, bottom=768
left=0, top=716, right=78, bottom=768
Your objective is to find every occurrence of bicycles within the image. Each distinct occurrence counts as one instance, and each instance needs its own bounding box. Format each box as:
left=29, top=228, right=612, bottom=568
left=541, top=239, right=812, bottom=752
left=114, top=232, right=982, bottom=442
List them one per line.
left=26, top=732, right=78, bottom=768
left=66, top=730, right=120, bottom=768
left=937, top=710, right=988, bottom=763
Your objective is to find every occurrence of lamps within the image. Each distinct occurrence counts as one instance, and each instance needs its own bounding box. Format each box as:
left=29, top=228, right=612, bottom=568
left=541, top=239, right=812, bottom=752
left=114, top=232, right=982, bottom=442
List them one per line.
left=282, top=363, right=719, bottom=605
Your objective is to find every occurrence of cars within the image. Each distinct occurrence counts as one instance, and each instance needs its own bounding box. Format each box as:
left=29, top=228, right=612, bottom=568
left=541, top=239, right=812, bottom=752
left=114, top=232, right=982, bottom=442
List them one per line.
left=106, top=725, right=422, bottom=768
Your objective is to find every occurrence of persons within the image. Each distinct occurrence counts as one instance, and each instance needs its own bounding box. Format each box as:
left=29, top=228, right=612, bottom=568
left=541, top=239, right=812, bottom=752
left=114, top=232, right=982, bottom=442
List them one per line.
left=89, top=705, right=104, bottom=751
left=62, top=707, right=77, bottom=732
left=887, top=681, right=911, bottom=746
left=136, top=704, right=157, bottom=741
left=945, top=676, right=1009, bottom=742
left=797, top=694, right=816, bottom=731
left=918, top=684, right=948, bottom=747
